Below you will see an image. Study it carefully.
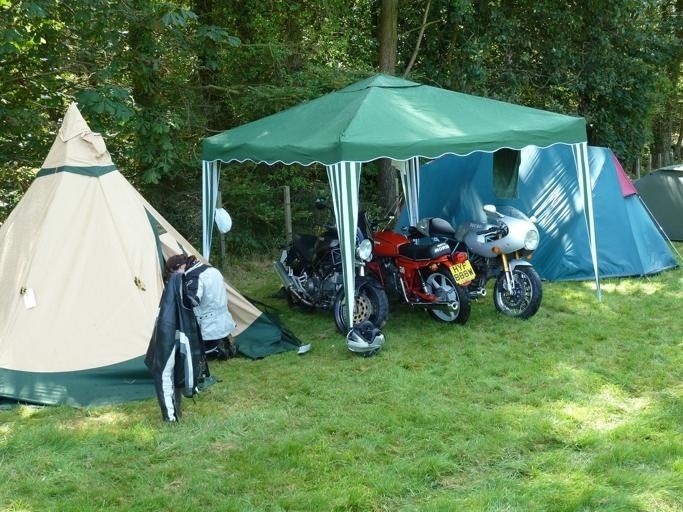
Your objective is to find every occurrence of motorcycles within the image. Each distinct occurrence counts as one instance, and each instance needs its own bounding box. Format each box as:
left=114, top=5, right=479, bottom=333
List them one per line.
left=272, top=198, right=543, bottom=340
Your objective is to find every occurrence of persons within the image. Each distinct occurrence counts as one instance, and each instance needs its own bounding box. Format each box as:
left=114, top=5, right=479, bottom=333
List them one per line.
left=164, top=253, right=238, bottom=360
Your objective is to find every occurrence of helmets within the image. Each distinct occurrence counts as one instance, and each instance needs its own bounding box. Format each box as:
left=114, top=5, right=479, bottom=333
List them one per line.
left=346, top=320, right=385, bottom=357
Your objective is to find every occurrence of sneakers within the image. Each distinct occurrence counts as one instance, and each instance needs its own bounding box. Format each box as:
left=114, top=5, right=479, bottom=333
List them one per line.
left=217, top=335, right=236, bottom=360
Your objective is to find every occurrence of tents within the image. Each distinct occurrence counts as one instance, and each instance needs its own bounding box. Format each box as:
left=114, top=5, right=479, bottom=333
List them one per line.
left=395, top=144, right=679, bottom=282
left=0, top=102, right=303, bottom=412
left=630, top=163, right=683, bottom=241
